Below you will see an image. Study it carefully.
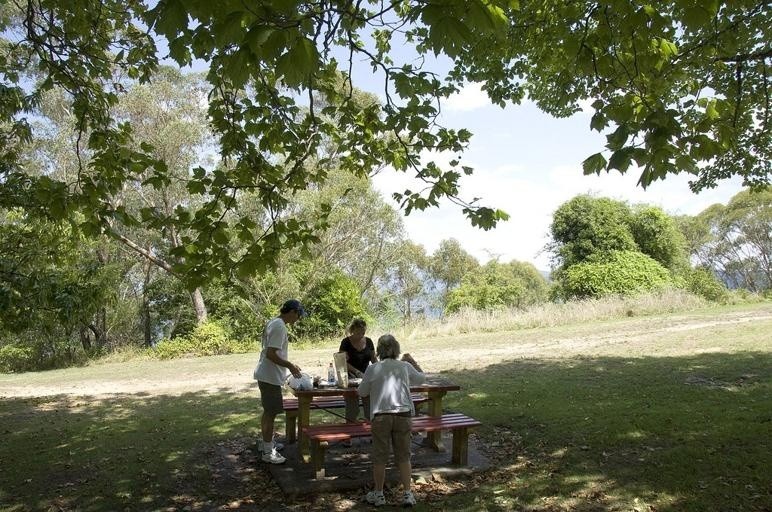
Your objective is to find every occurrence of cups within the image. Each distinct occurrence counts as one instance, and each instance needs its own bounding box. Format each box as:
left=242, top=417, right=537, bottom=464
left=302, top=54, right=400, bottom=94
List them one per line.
left=333, top=351, right=348, bottom=388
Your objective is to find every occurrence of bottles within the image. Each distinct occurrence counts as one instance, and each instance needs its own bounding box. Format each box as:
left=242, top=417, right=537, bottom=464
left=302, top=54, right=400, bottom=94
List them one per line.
left=328, top=363, right=335, bottom=382
left=314, top=376, right=321, bottom=388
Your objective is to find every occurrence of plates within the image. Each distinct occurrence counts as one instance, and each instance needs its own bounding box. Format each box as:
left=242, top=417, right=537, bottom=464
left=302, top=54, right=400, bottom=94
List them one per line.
left=349, top=378, right=362, bottom=386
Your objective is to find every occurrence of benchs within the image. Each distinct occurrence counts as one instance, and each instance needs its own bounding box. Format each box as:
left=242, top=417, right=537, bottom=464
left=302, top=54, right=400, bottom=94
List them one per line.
left=281, top=392, right=482, bottom=481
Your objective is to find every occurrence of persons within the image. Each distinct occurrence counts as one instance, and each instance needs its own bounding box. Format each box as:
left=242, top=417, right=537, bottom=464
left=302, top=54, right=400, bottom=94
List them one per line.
left=357, top=333, right=427, bottom=506
left=339, top=318, right=379, bottom=448
left=254, top=299, right=308, bottom=464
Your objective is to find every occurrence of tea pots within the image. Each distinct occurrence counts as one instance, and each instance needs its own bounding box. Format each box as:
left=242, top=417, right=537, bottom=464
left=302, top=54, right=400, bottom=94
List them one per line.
left=288, top=372, right=313, bottom=390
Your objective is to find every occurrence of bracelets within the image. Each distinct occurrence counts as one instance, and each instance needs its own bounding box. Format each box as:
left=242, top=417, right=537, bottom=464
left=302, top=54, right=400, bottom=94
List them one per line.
left=408, top=359, right=414, bottom=363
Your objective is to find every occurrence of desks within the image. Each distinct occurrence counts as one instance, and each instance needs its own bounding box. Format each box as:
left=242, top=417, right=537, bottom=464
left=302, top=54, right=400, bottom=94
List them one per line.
left=291, top=375, right=461, bottom=464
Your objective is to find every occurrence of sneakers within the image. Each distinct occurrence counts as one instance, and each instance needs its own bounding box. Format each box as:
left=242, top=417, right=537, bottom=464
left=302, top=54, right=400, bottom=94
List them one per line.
left=401, top=490, right=417, bottom=507
left=258, top=440, right=284, bottom=452
left=366, top=491, right=386, bottom=506
left=262, top=448, right=287, bottom=465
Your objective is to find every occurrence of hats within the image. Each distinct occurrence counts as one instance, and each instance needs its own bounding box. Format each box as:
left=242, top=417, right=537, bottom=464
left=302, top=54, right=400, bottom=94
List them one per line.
left=282, top=299, right=309, bottom=317
